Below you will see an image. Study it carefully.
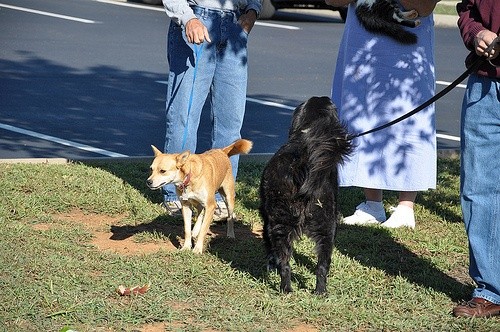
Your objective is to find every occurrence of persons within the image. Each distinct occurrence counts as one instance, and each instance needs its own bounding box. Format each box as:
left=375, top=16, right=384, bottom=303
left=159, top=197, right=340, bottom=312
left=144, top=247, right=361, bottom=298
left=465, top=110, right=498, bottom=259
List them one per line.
left=162, top=1, right=276, bottom=220
left=453, top=0, right=500, bottom=318
left=327, top=0, right=443, bottom=228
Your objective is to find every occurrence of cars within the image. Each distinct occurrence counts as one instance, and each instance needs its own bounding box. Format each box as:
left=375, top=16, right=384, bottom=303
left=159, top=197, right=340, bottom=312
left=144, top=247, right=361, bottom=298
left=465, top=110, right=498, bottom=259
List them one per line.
left=127, top=0, right=350, bottom=24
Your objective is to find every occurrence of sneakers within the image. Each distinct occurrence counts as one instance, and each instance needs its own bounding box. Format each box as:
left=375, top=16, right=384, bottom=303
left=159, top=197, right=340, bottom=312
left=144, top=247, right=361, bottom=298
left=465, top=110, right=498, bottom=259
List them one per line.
left=379, top=204, right=416, bottom=228
left=342, top=200, right=387, bottom=226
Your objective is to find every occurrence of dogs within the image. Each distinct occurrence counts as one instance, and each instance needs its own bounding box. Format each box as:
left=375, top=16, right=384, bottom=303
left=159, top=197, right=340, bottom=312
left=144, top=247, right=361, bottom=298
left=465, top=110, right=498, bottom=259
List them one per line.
left=146, top=139, right=253, bottom=255
left=258, top=95, right=359, bottom=296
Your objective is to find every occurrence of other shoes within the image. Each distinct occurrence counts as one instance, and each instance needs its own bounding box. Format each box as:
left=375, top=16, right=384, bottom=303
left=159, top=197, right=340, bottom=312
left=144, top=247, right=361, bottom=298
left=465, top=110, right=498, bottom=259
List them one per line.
left=213, top=200, right=238, bottom=224
left=163, top=200, right=184, bottom=216
left=452, top=297, right=500, bottom=318
